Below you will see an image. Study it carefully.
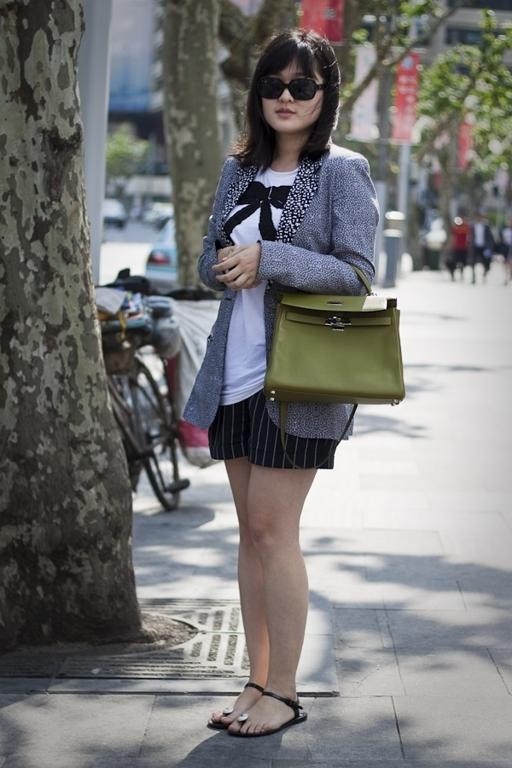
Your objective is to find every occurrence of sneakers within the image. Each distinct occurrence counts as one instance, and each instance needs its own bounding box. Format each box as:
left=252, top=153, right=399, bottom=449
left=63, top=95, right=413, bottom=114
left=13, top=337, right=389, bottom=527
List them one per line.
left=450, top=271, right=512, bottom=287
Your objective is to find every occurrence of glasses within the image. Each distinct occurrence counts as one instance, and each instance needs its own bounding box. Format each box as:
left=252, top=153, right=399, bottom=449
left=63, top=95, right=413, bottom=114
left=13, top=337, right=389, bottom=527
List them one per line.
left=256, top=76, right=327, bottom=101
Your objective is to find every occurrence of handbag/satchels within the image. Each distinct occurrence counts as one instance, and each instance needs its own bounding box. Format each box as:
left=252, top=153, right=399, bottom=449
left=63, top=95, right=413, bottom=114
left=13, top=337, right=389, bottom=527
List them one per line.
left=262, top=263, right=407, bottom=470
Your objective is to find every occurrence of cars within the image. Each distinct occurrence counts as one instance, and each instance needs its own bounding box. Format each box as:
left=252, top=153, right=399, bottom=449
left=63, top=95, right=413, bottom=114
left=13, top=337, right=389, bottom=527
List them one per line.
left=422, top=218, right=454, bottom=271
left=100, top=196, right=131, bottom=229
left=142, top=199, right=177, bottom=232
left=144, top=219, right=188, bottom=301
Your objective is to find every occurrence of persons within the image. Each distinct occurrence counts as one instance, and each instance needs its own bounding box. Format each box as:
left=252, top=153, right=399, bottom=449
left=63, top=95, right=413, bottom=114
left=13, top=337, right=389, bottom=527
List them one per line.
left=182, top=28, right=379, bottom=739
left=443, top=207, right=512, bottom=288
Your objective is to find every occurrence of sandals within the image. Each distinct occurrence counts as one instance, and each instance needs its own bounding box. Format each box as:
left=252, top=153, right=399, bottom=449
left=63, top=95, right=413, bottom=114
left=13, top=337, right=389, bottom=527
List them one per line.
left=227, top=690, right=307, bottom=738
left=207, top=682, right=265, bottom=729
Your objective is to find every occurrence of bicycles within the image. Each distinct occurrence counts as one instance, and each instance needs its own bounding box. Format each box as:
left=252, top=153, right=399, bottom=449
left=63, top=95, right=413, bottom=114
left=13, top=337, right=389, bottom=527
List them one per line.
left=93, top=266, right=192, bottom=516
